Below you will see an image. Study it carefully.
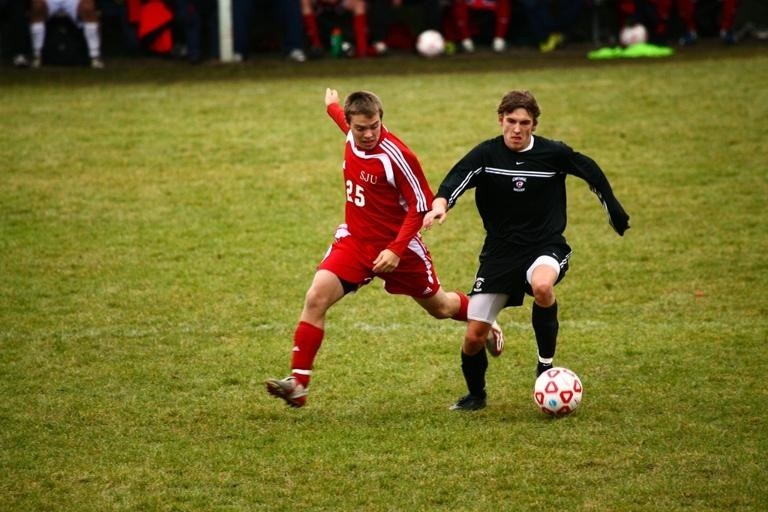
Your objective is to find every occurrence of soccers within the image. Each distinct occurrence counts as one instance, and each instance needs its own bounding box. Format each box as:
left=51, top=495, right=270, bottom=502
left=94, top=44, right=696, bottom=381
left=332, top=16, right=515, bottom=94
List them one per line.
left=416, top=31, right=444, bottom=58
left=621, top=24, right=646, bottom=46
left=534, top=368, right=583, bottom=417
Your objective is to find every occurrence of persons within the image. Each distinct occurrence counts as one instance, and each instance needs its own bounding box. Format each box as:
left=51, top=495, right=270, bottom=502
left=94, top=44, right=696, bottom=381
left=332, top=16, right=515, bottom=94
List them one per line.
left=422, top=91, right=630, bottom=412
left=261, top=88, right=504, bottom=406
left=0, top=1, right=767, bottom=71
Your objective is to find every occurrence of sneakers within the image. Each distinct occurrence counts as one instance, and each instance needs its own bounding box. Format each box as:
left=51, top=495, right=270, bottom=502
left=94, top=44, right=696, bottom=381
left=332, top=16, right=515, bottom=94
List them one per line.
left=448, top=392, right=486, bottom=411
left=266, top=376, right=310, bottom=408
left=484, top=320, right=504, bottom=357
